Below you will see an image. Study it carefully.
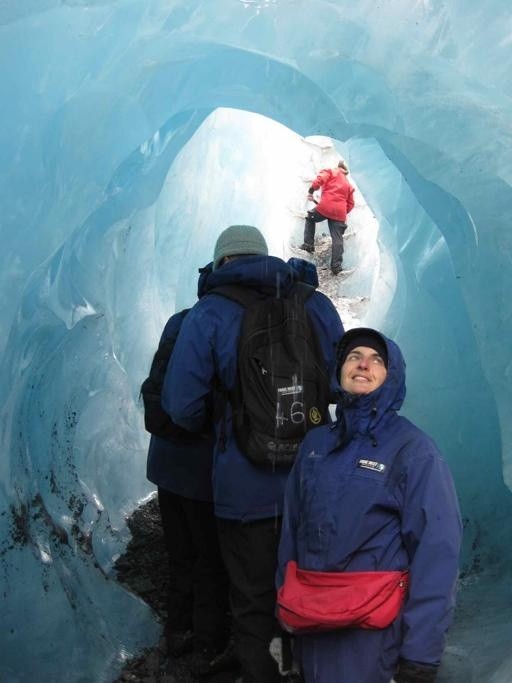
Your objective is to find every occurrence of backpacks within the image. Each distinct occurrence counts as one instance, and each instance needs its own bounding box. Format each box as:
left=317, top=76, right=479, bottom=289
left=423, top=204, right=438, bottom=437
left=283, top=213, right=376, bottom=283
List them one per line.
left=210, top=282, right=333, bottom=473
left=140, top=310, right=210, bottom=440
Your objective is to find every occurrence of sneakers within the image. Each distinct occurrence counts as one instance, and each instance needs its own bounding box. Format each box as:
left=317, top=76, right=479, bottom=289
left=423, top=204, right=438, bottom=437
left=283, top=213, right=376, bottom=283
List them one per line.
left=333, top=266, right=342, bottom=273
left=301, top=244, right=314, bottom=252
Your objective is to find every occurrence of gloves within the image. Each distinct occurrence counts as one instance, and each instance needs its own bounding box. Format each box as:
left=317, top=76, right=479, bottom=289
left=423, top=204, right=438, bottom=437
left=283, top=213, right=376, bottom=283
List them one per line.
left=387, top=660, right=439, bottom=683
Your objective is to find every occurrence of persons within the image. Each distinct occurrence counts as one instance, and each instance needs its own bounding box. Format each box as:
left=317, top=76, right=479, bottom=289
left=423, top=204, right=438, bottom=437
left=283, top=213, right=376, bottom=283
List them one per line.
left=138, top=261, right=230, bottom=665
left=274, top=326, right=463, bottom=683
left=300, top=160, right=355, bottom=277
left=159, top=224, right=349, bottom=682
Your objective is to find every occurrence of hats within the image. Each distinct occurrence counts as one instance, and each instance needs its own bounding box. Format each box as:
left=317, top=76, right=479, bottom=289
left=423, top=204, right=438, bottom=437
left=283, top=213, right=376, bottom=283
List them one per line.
left=339, top=334, right=388, bottom=368
left=212, top=225, right=269, bottom=273
left=338, top=164, right=348, bottom=173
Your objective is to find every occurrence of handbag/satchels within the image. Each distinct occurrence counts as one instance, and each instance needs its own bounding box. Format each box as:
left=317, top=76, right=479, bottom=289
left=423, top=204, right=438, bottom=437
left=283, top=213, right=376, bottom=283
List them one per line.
left=274, top=561, right=408, bottom=636
